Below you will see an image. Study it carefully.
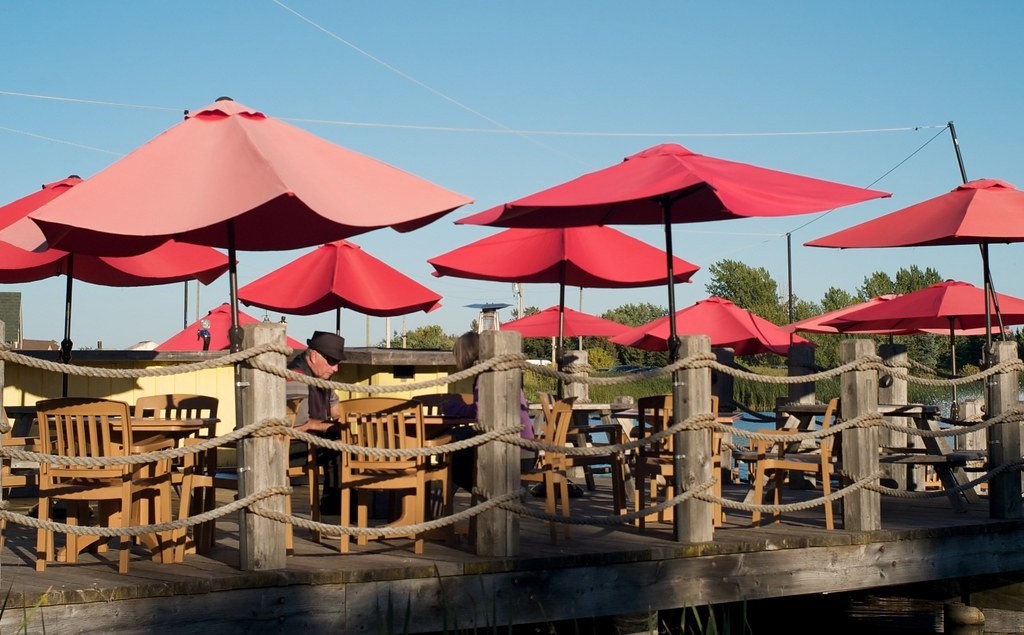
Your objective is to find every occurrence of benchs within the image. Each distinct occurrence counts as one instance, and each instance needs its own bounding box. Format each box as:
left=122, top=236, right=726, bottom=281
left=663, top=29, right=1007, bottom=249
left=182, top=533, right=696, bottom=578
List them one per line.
left=734, top=440, right=991, bottom=519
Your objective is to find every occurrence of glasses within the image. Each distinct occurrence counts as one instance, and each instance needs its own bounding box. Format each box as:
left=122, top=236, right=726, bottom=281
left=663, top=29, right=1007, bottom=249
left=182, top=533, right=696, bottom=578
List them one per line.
left=312, top=349, right=341, bottom=366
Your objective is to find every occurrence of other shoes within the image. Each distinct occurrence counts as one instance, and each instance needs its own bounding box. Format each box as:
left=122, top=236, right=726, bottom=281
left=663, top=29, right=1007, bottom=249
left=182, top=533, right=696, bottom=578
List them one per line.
left=321, top=533, right=341, bottom=539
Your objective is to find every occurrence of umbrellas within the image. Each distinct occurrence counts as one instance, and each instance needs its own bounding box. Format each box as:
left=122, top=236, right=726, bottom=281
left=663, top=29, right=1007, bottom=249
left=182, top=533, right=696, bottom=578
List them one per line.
left=0, top=96, right=1024, bottom=468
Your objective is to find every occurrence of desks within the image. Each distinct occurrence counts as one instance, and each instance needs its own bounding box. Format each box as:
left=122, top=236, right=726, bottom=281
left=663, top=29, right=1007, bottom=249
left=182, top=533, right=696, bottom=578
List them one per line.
left=654, top=414, right=734, bottom=519
left=343, top=411, right=478, bottom=531
left=528, top=400, right=643, bottom=502
left=743, top=408, right=978, bottom=520
left=34, top=413, right=219, bottom=550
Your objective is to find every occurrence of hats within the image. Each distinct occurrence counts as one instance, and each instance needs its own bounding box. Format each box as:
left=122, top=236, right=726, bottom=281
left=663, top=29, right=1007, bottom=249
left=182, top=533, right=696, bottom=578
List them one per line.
left=307, top=331, right=347, bottom=360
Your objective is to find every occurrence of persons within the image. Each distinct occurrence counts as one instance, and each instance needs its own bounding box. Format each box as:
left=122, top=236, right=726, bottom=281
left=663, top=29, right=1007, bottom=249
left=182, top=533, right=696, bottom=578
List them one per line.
left=440, top=331, right=539, bottom=475
left=233, top=331, right=358, bottom=516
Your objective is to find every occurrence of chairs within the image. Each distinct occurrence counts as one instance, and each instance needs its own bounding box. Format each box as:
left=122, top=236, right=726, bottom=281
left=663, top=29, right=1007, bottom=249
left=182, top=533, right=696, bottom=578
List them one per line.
left=3, top=397, right=838, bottom=571
